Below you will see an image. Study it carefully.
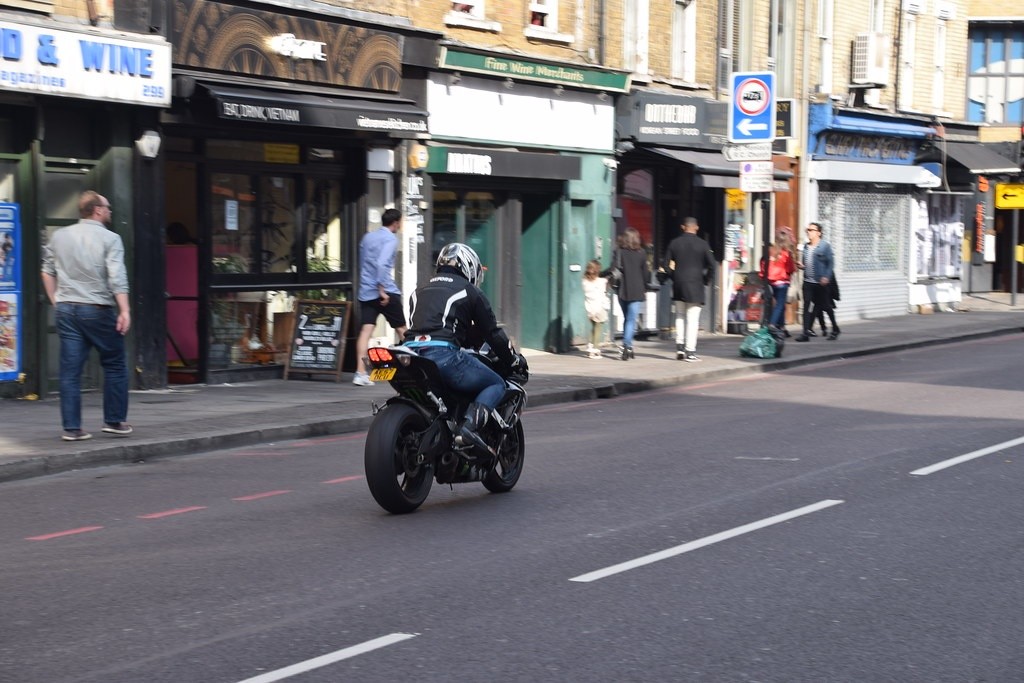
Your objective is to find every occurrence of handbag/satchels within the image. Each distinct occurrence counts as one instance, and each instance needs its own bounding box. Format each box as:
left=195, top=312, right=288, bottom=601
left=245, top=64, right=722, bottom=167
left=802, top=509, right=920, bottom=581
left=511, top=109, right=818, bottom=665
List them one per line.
left=610, top=268, right=624, bottom=291
left=740, top=324, right=785, bottom=359
left=763, top=285, right=774, bottom=301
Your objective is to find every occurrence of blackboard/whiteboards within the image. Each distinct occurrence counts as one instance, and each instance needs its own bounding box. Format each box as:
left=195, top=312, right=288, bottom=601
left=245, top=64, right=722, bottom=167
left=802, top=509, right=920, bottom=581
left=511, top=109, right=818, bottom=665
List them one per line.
left=208, top=299, right=267, bottom=364
left=286, top=300, right=356, bottom=374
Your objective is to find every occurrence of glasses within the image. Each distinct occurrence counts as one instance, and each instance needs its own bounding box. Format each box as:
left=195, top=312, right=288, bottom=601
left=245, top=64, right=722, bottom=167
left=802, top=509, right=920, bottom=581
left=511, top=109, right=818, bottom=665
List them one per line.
left=97, top=204, right=112, bottom=211
left=807, top=229, right=818, bottom=232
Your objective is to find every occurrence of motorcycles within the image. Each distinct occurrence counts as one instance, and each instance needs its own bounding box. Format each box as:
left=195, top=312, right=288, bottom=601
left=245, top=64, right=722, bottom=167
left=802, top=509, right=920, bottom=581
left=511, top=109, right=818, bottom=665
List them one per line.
left=361, top=346, right=530, bottom=515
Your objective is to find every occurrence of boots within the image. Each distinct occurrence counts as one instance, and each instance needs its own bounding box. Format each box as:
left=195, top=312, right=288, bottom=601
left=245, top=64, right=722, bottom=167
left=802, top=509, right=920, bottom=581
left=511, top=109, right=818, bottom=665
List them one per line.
left=461, top=400, right=496, bottom=460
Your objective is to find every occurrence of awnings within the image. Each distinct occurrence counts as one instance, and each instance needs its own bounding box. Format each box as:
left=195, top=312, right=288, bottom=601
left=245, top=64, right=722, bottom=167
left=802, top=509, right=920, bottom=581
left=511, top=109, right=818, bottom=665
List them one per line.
left=926, top=140, right=1022, bottom=174
left=638, top=145, right=794, bottom=195
left=191, top=81, right=430, bottom=136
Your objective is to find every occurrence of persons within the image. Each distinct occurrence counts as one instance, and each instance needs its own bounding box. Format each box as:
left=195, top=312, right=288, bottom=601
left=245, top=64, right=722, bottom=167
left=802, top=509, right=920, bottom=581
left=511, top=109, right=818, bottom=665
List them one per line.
left=599, top=227, right=651, bottom=359
left=759, top=228, right=798, bottom=337
left=400, top=242, right=527, bottom=461
left=793, top=222, right=841, bottom=342
left=580, top=260, right=613, bottom=358
left=41, top=191, right=133, bottom=440
left=661, top=217, right=715, bottom=362
left=352, top=208, right=408, bottom=387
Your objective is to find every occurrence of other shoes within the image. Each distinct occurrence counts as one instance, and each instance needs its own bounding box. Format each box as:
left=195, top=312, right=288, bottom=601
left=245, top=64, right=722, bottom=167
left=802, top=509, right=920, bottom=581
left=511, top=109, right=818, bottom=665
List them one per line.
left=686, top=355, right=701, bottom=362
left=588, top=346, right=601, bottom=359
left=677, top=344, right=684, bottom=359
left=829, top=330, right=840, bottom=339
left=622, top=345, right=634, bottom=360
left=794, top=334, right=809, bottom=342
left=822, top=329, right=826, bottom=336
left=807, top=329, right=817, bottom=337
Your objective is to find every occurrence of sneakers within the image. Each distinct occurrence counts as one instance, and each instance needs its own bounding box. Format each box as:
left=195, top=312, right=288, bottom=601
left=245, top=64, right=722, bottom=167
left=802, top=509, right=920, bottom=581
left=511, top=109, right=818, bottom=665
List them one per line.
left=62, top=428, right=92, bottom=440
left=353, top=371, right=375, bottom=386
left=100, top=421, right=132, bottom=435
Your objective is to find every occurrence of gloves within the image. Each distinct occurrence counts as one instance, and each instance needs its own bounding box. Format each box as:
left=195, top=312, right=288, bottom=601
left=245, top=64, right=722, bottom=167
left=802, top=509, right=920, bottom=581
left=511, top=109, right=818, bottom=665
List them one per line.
left=517, top=352, right=531, bottom=372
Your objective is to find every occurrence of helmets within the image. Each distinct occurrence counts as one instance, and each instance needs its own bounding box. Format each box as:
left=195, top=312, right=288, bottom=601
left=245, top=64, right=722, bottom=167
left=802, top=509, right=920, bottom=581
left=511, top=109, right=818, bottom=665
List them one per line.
left=435, top=242, right=484, bottom=287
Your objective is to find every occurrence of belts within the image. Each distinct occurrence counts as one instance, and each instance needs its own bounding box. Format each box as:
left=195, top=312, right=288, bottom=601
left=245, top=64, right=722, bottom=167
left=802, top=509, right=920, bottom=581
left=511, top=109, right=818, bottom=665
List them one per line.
left=64, top=301, right=111, bottom=307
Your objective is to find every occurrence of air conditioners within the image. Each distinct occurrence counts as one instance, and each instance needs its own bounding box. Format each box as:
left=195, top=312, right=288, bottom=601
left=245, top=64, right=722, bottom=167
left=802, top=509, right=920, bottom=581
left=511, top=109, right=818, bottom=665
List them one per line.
left=851, top=31, right=890, bottom=87
